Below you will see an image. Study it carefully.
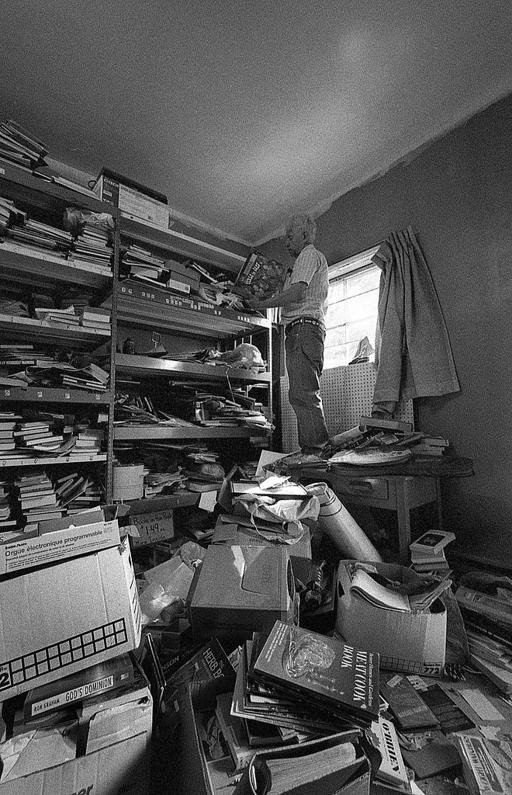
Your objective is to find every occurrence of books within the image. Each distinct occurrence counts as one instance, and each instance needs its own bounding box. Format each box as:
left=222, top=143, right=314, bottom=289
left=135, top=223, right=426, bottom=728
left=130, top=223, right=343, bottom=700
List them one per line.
left=234, top=252, right=289, bottom=305
left=1, top=414, right=512, bottom=793
left=0, top=116, right=277, bottom=535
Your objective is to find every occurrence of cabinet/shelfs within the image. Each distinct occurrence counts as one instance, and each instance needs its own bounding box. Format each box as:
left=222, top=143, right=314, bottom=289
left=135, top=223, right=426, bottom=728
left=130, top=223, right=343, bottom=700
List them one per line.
left=0, top=158, right=272, bottom=595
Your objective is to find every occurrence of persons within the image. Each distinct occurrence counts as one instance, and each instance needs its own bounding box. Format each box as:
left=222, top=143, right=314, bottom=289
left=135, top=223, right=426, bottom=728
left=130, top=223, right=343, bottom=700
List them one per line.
left=247, top=213, right=329, bottom=462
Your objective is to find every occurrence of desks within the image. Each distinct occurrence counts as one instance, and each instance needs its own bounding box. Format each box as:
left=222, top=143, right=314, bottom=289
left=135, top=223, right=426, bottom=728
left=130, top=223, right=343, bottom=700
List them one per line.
left=287, top=468, right=441, bottom=560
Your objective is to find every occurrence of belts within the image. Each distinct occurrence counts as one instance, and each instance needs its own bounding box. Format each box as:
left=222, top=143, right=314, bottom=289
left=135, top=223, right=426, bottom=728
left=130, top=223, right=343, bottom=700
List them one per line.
left=285, top=317, right=327, bottom=334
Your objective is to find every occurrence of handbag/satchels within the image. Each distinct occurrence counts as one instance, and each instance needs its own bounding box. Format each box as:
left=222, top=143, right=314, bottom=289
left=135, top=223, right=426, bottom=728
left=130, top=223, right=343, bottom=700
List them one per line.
left=232, top=493, right=320, bottom=523
